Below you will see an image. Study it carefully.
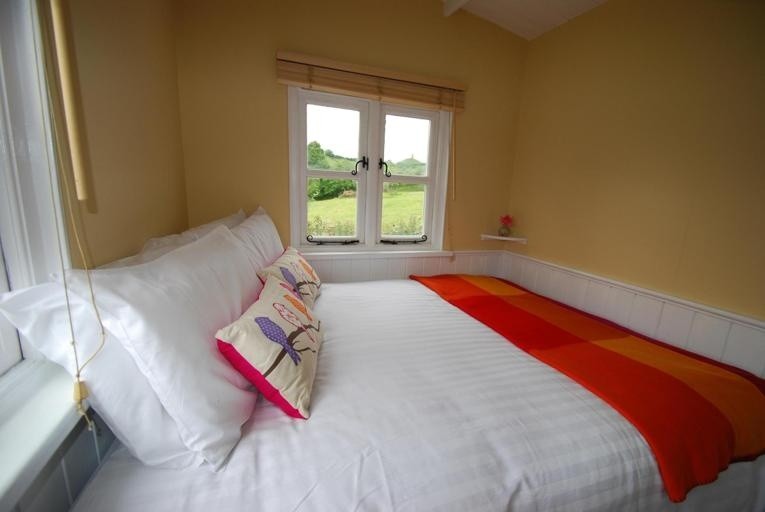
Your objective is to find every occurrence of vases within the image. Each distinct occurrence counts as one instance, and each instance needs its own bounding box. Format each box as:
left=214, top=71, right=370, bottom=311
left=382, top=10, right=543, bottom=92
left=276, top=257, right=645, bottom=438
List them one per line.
left=497, top=226, right=511, bottom=238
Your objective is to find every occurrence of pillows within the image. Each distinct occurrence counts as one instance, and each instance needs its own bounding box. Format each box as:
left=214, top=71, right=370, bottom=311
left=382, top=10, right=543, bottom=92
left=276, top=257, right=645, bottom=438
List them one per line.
left=144, top=207, right=286, bottom=267
left=0, top=244, right=205, bottom=473
left=213, top=272, right=323, bottom=422
left=256, top=244, right=322, bottom=312
left=50, top=227, right=265, bottom=472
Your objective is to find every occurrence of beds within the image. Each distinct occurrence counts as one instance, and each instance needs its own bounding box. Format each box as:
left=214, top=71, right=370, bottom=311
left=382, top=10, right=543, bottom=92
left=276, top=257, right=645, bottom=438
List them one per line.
left=1, top=204, right=765, bottom=512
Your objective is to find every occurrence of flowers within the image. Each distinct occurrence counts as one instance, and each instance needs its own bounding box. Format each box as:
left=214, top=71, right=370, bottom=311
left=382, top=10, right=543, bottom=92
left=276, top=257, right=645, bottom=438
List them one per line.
left=497, top=214, right=516, bottom=227
